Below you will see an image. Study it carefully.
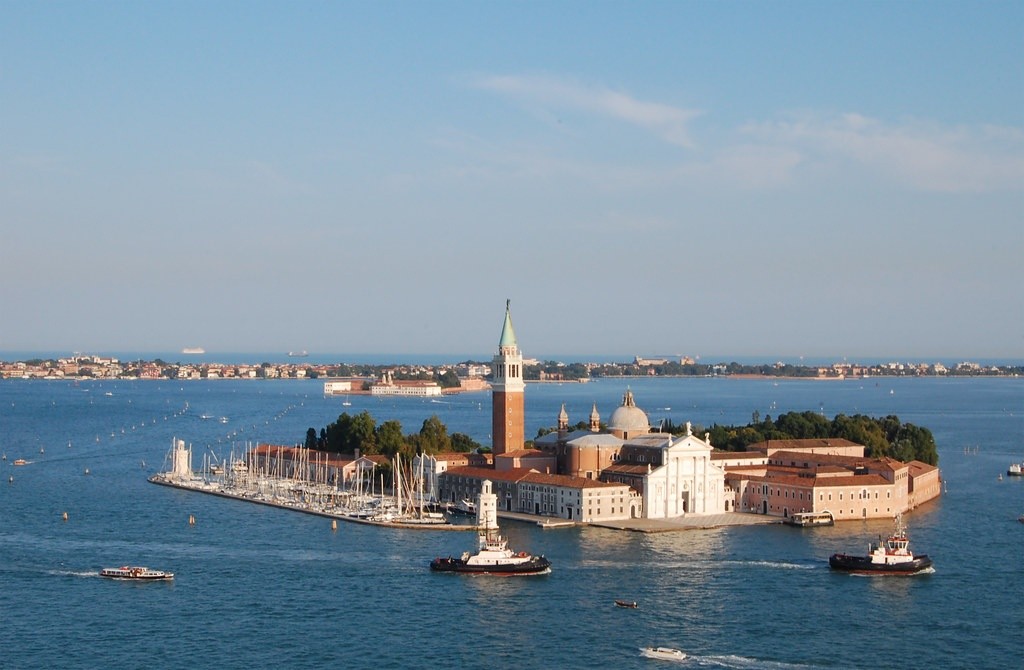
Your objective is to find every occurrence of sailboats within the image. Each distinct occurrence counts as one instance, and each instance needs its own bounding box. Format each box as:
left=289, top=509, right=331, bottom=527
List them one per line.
left=146, top=437, right=448, bottom=527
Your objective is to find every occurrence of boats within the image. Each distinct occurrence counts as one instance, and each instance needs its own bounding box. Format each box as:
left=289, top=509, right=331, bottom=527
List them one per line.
left=783, top=512, right=833, bottom=528
left=100, top=566, right=175, bottom=579
left=615, top=599, right=638, bottom=607
left=829, top=510, right=933, bottom=573
left=13, top=458, right=26, bottom=465
left=1008, top=461, right=1024, bottom=477
left=430, top=511, right=553, bottom=572
